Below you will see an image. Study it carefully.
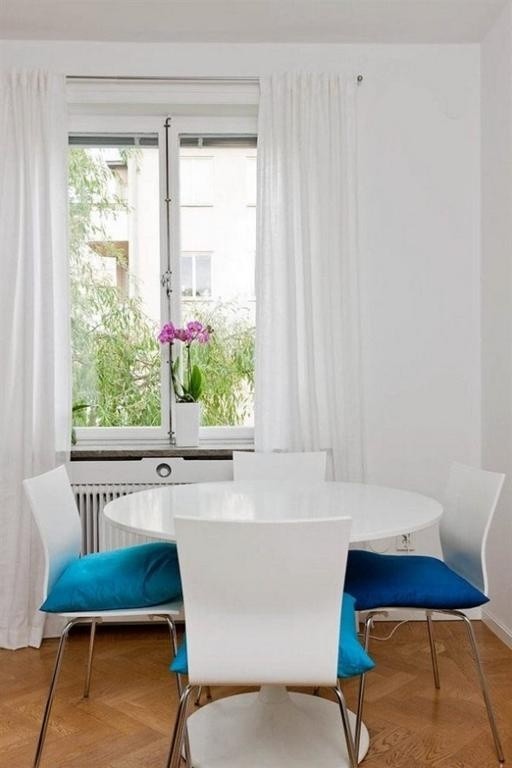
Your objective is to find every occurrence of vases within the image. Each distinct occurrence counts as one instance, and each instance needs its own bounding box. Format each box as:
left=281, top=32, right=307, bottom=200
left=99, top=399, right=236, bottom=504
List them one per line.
left=172, top=403, right=202, bottom=447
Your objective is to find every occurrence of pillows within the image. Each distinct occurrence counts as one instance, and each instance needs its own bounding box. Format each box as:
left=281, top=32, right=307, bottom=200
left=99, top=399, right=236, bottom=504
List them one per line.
left=352, top=551, right=488, bottom=611
left=38, top=541, right=180, bottom=612
left=167, top=594, right=377, bottom=676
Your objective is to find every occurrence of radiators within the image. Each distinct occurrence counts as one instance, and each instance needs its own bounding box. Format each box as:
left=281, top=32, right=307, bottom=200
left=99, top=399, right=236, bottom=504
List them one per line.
left=70, top=459, right=233, bottom=556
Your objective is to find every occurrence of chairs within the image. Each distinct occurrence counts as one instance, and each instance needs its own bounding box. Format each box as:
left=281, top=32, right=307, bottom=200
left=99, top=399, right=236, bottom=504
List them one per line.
left=165, top=516, right=359, bottom=765
left=233, top=450, right=328, bottom=481
left=347, top=469, right=503, bottom=762
left=24, top=466, right=184, bottom=768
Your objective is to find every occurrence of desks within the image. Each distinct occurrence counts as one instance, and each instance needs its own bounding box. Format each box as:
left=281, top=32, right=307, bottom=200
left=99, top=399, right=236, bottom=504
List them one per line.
left=104, top=479, right=444, bottom=767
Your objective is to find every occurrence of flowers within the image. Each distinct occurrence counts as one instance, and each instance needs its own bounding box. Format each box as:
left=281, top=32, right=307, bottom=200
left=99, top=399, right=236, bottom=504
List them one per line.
left=156, top=321, right=212, bottom=403
